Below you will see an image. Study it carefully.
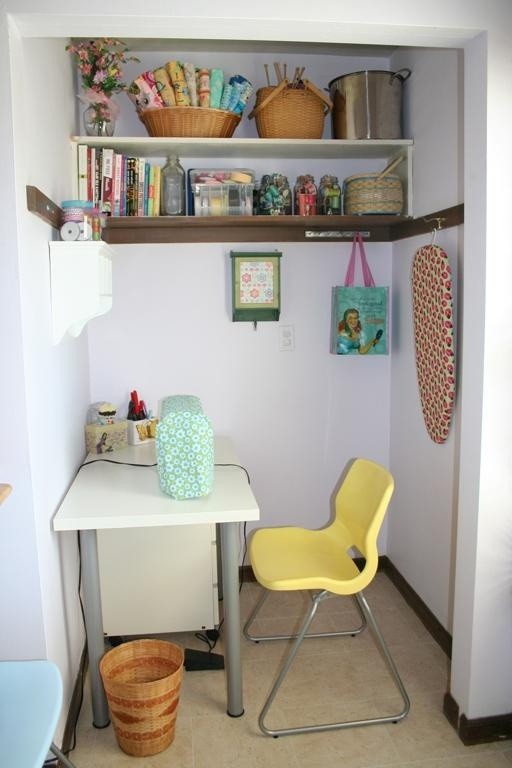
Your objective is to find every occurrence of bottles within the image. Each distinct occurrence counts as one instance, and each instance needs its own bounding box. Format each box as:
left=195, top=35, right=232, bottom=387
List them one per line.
left=257, top=173, right=292, bottom=215
left=316, top=174, right=343, bottom=215
left=58, top=198, right=101, bottom=240
left=292, top=174, right=316, bottom=216
left=160, top=154, right=186, bottom=217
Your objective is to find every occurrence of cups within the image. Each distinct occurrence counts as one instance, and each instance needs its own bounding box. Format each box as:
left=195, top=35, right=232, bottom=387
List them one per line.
left=127, top=418, right=148, bottom=444
left=147, top=418, right=159, bottom=438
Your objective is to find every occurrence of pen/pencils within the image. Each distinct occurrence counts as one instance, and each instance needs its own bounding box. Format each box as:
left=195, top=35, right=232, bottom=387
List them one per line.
left=128, top=391, right=146, bottom=420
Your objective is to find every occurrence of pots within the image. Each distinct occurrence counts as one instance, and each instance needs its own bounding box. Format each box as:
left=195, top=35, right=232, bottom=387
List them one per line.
left=320, top=67, right=413, bottom=141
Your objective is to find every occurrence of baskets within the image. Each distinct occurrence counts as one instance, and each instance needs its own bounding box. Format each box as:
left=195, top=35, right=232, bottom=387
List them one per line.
left=345, top=154, right=405, bottom=218
left=247, top=79, right=334, bottom=139
left=137, top=105, right=241, bottom=138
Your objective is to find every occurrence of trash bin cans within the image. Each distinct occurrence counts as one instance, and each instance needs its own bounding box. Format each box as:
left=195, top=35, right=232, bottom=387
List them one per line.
left=99, top=638, right=184, bottom=756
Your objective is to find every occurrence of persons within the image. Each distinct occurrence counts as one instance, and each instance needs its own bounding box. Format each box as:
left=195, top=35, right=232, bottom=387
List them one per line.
left=338, top=307, right=384, bottom=357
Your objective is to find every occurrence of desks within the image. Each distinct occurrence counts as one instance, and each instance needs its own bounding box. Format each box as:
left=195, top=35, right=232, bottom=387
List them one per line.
left=0, top=483, right=11, bottom=504
left=53, top=437, right=260, bottom=727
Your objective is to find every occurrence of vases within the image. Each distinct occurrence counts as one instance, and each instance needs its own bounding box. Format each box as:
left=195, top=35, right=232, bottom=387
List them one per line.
left=82, top=103, right=118, bottom=136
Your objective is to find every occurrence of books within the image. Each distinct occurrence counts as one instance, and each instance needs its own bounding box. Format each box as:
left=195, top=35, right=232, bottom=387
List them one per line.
left=78, top=142, right=163, bottom=218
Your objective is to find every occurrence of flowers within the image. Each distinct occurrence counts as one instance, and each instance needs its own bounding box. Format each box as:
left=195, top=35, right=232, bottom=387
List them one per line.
left=64, top=37, right=141, bottom=137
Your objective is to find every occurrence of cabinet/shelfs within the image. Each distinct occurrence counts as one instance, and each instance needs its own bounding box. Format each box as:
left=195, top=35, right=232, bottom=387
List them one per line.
left=67, top=135, right=412, bottom=240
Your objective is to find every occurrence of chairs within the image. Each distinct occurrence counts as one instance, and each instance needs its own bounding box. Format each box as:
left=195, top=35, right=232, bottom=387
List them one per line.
left=0, top=660, right=76, bottom=768
left=242, top=458, right=411, bottom=736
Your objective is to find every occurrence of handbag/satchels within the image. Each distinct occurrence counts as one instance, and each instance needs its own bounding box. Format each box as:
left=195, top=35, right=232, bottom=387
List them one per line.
left=330, top=286, right=390, bottom=356
